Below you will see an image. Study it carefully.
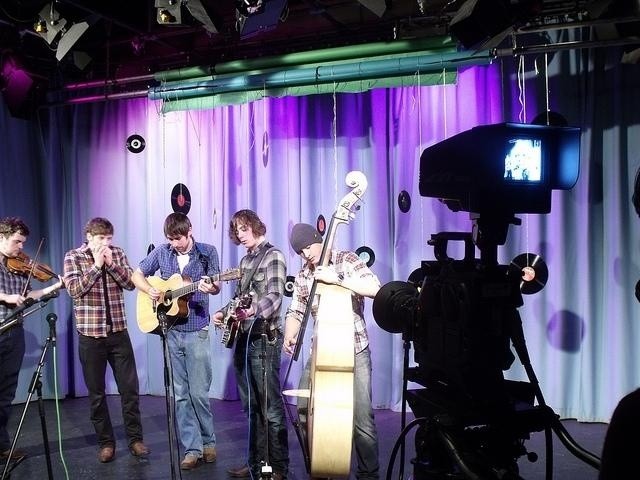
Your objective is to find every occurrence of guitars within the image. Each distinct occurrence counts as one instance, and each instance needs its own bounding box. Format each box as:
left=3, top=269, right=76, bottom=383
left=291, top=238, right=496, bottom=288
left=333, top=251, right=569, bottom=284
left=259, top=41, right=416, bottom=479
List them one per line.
left=136, top=266, right=243, bottom=334
left=221, top=294, right=254, bottom=348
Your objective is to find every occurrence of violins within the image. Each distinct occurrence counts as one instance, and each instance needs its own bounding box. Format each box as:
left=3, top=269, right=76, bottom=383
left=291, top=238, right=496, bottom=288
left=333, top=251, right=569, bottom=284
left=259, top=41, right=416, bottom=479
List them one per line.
left=8, top=252, right=62, bottom=282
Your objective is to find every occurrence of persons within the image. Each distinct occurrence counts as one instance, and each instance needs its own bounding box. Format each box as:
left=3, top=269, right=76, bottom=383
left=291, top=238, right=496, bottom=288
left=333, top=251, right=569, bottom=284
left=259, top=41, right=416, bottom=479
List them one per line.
left=0, top=217, right=64, bottom=466
left=506, top=162, right=530, bottom=180
left=599, top=165, right=640, bottom=479
left=130, top=212, right=221, bottom=470
left=283, top=223, right=382, bottom=480
left=64, top=216, right=152, bottom=463
left=211, top=209, right=289, bottom=480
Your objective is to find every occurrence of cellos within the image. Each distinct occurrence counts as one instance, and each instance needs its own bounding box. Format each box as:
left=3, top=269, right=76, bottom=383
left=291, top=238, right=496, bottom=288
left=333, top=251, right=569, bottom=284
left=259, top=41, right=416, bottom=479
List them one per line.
left=305, top=172, right=367, bottom=478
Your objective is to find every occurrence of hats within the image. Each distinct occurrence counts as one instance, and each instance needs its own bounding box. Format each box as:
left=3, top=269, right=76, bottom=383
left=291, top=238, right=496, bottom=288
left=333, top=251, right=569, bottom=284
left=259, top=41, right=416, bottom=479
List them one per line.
left=291, top=223, right=322, bottom=255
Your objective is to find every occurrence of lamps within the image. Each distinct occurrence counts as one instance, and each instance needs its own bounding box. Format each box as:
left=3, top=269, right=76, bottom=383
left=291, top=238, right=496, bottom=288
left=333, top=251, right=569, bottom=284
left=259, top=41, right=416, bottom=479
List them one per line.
left=153, top=1, right=220, bottom=35
left=234, top=0, right=290, bottom=41
left=19, top=0, right=95, bottom=62
left=449, top=0, right=520, bottom=50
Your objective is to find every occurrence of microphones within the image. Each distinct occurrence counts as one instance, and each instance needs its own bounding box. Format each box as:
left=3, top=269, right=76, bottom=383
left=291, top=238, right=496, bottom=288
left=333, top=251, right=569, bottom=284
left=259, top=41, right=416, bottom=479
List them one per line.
left=157, top=309, right=171, bottom=337
left=45, top=312, right=58, bottom=347
left=260, top=315, right=279, bottom=347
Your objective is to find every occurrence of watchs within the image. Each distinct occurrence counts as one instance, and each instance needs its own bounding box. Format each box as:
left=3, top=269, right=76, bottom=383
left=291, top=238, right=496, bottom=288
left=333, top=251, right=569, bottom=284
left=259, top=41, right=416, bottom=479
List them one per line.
left=336, top=272, right=345, bottom=287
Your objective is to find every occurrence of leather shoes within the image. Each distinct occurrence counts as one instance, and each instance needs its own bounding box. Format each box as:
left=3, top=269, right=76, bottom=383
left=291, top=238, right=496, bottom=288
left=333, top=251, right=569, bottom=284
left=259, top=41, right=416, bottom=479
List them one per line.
left=180, top=455, right=198, bottom=469
left=101, top=447, right=115, bottom=462
left=130, top=442, right=150, bottom=456
left=270, top=473, right=283, bottom=480
left=228, top=466, right=250, bottom=478
left=203, top=447, right=216, bottom=463
left=0, top=449, right=26, bottom=459
